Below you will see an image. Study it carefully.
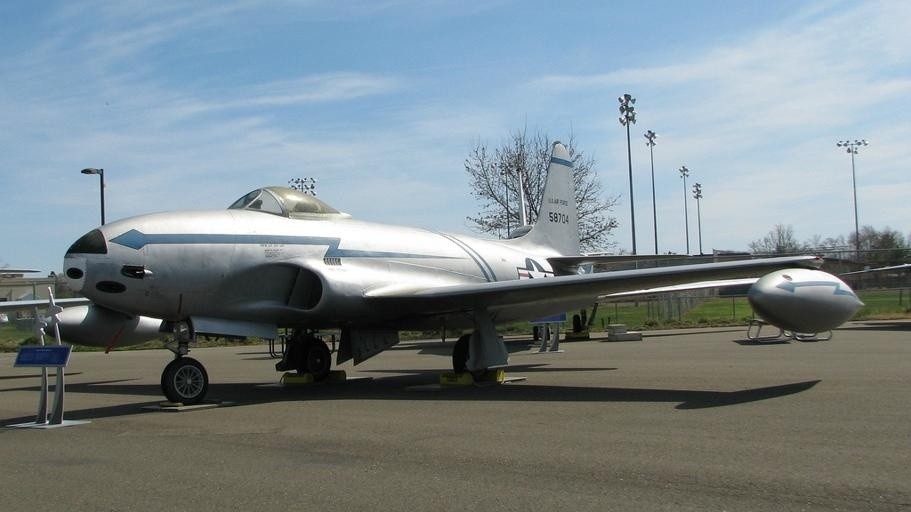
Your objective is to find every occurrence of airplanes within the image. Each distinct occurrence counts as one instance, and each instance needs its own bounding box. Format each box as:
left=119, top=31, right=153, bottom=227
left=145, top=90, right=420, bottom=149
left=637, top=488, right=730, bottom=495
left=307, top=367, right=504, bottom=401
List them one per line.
left=0, top=184, right=873, bottom=407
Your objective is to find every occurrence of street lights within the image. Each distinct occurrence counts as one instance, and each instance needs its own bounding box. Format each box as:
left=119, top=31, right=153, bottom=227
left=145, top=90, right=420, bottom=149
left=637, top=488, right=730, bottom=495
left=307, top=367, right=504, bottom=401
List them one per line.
left=691, top=181, right=701, bottom=255
left=834, top=137, right=870, bottom=261
left=614, top=89, right=636, bottom=255
left=642, top=128, right=658, bottom=255
left=677, top=165, right=689, bottom=256
left=287, top=175, right=317, bottom=198
left=79, top=167, right=104, bottom=227
left=488, top=158, right=515, bottom=239
